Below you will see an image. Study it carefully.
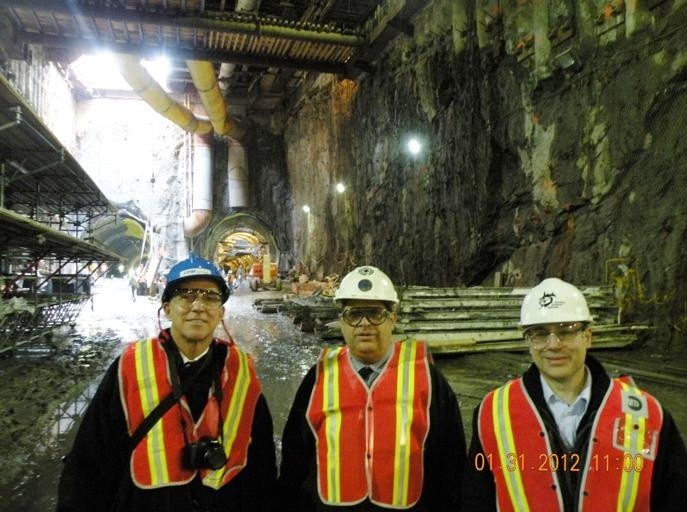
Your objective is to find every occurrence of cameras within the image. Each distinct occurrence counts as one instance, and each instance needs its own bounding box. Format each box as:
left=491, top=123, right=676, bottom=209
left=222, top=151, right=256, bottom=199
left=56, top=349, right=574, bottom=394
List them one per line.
left=182, top=440, right=226, bottom=470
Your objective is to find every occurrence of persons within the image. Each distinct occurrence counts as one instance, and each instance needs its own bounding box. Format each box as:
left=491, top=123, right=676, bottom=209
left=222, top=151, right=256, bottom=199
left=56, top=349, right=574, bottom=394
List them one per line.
left=467, top=275, right=686, bottom=510
left=55, top=252, right=278, bottom=509
left=128, top=275, right=137, bottom=301
left=281, top=262, right=466, bottom=512
left=138, top=276, right=146, bottom=295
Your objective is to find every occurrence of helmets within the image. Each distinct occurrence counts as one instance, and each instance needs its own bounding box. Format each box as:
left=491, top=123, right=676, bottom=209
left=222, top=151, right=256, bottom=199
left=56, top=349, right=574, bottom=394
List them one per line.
left=332, top=265, right=399, bottom=310
left=516, top=276, right=593, bottom=329
left=160, top=250, right=227, bottom=306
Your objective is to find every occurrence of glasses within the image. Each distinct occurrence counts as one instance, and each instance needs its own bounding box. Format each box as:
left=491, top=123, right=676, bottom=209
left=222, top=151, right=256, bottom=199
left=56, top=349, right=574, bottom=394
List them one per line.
left=167, top=288, right=224, bottom=308
left=521, top=322, right=588, bottom=347
left=336, top=306, right=388, bottom=327
